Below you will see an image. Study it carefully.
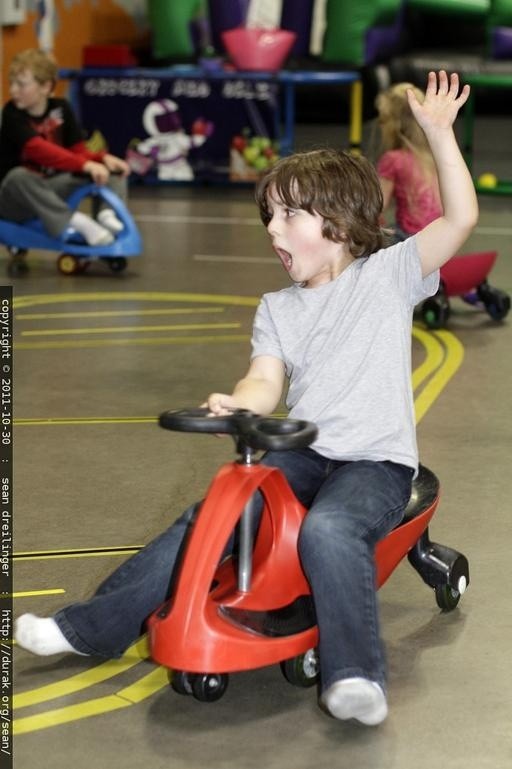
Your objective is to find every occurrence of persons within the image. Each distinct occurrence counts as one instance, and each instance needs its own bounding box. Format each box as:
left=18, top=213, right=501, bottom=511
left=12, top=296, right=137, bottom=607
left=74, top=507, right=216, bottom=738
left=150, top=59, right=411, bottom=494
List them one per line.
left=0, top=45, right=133, bottom=249
left=369, top=80, right=444, bottom=245
left=13, top=69, right=484, bottom=729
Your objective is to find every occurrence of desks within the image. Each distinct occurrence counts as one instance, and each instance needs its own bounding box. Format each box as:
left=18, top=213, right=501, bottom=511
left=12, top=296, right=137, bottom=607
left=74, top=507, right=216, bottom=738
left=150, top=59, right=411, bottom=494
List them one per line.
left=58, top=65, right=365, bottom=190
